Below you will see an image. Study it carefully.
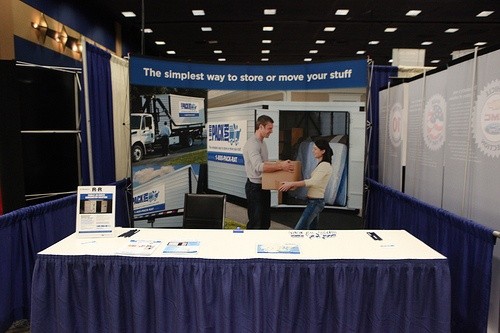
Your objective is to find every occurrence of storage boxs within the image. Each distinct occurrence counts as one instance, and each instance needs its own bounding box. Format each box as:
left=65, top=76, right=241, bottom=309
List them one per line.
left=262, top=160, right=303, bottom=191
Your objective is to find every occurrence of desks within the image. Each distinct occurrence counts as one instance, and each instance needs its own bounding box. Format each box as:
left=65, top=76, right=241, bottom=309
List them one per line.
left=31, top=227, right=452, bottom=333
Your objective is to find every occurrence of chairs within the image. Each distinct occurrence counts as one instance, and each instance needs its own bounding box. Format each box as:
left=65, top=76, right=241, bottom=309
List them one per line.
left=184, top=193, right=226, bottom=229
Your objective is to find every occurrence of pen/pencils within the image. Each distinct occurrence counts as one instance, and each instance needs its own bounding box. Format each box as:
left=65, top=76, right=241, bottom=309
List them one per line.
left=118, top=229, right=140, bottom=238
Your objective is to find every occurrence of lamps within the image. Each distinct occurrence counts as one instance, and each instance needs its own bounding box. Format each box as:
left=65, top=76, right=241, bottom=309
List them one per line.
left=55, top=23, right=68, bottom=52
left=72, top=33, right=82, bottom=61
left=31, top=12, right=48, bottom=43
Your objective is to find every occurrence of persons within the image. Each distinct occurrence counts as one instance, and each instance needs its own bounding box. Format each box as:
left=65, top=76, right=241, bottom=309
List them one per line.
left=159, top=121, right=171, bottom=157
left=276, top=137, right=333, bottom=229
left=241, top=115, right=294, bottom=229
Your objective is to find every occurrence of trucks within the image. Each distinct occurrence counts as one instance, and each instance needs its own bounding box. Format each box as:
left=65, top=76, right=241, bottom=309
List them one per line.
left=131, top=94, right=206, bottom=161
